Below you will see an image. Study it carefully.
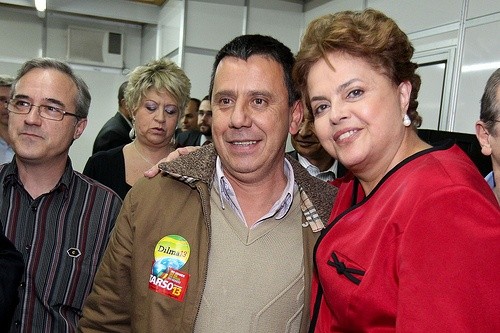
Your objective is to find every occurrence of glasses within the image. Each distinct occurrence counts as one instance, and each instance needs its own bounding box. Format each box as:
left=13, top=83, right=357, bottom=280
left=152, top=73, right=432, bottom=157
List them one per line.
left=4, top=98, right=81, bottom=121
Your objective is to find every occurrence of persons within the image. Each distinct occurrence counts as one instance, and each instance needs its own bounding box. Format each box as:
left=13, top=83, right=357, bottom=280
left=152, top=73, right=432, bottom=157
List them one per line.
left=144, top=8, right=500, bottom=333
left=0, top=58, right=124, bottom=333
left=81, top=59, right=212, bottom=201
left=283, top=94, right=348, bottom=185
left=76, top=33, right=338, bottom=333
left=475, top=68, right=500, bottom=205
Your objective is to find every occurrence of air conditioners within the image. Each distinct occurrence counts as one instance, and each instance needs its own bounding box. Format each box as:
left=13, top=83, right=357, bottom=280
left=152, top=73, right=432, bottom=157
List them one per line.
left=64, top=24, right=125, bottom=70
